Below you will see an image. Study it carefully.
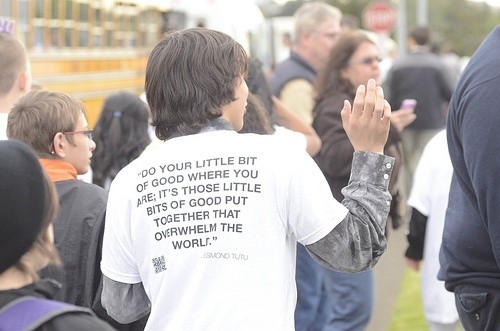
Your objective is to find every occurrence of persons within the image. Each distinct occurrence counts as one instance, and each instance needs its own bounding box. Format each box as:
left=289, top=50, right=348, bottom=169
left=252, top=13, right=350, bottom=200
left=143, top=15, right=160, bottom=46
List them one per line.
left=98, top=26, right=396, bottom=330
left=436, top=25, right=500, bottom=330
left=1, top=33, right=32, bottom=140
left=0, top=138, right=117, bottom=331
left=244, top=0, right=456, bottom=231
left=78, top=90, right=151, bottom=192
left=405, top=127, right=469, bottom=331
left=7, top=88, right=151, bottom=331
left=238, top=91, right=372, bottom=331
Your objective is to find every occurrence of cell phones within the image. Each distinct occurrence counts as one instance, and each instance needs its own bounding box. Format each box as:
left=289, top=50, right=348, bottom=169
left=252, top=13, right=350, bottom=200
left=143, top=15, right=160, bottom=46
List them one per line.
left=401, top=99, right=417, bottom=110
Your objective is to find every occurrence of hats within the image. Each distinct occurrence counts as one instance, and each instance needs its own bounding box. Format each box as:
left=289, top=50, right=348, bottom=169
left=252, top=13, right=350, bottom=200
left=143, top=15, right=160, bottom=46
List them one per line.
left=0, top=140, right=44, bottom=273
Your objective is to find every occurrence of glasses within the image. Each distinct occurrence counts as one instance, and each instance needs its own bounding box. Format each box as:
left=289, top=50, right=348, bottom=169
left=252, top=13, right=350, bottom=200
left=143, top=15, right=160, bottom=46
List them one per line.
left=350, top=56, right=382, bottom=66
left=51, top=129, right=94, bottom=155
